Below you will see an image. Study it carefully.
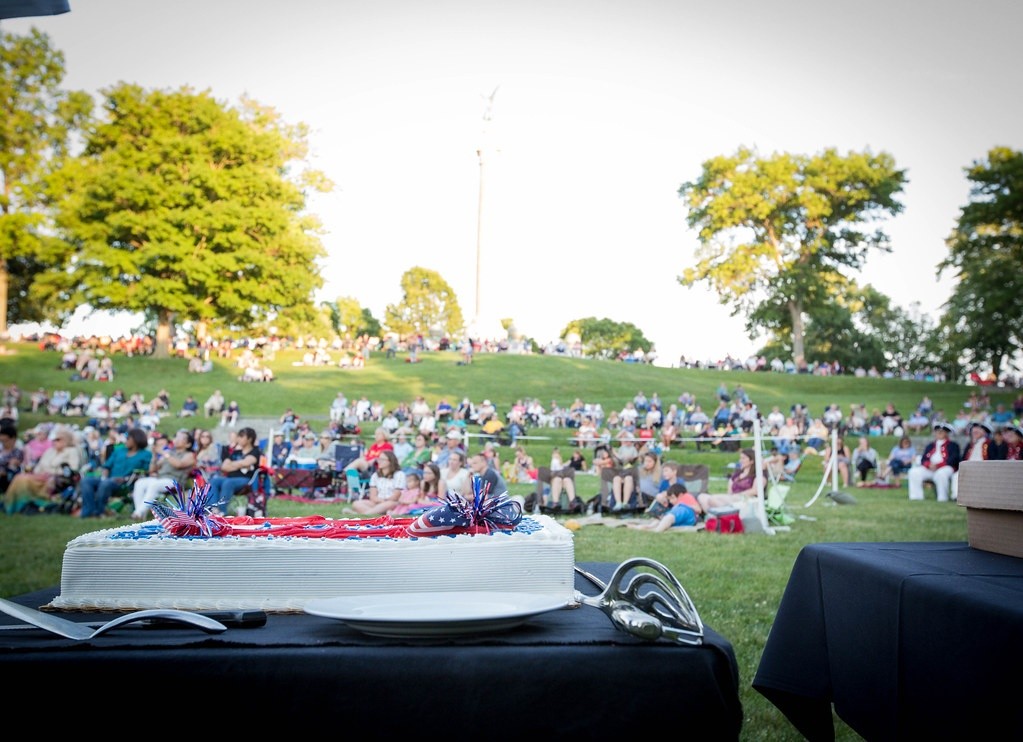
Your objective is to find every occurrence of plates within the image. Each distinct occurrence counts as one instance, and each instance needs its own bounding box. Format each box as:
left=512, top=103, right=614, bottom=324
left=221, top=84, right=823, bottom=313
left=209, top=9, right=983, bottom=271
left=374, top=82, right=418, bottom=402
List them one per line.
left=304, top=592, right=568, bottom=638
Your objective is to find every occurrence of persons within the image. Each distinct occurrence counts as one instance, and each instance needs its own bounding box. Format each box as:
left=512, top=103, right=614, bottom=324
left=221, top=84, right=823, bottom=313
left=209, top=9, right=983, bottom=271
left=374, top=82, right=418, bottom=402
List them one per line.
left=618, top=345, right=657, bottom=366
left=0, top=408, right=360, bottom=519
left=398, top=332, right=532, bottom=363
left=177, top=333, right=398, bottom=382
left=854, top=365, right=944, bottom=382
left=40, top=330, right=156, bottom=382
left=543, top=339, right=581, bottom=357
left=0, top=383, right=239, bottom=427
left=329, top=381, right=1022, bottom=533
left=680, top=355, right=844, bottom=375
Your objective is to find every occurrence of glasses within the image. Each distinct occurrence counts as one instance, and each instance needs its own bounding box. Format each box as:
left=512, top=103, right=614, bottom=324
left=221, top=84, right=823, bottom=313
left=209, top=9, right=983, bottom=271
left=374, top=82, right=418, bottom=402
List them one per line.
left=52, top=439, right=61, bottom=442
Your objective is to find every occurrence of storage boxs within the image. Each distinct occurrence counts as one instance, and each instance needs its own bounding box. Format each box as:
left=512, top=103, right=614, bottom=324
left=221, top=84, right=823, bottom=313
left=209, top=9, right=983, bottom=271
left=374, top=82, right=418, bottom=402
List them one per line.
left=956, top=459, right=1023, bottom=558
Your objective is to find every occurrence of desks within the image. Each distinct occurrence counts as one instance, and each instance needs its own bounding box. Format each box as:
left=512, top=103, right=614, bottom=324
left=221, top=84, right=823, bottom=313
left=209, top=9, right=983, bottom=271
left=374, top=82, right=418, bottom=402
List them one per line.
left=750, top=542, right=1023, bottom=742
left=274, top=469, right=333, bottom=497
left=0, top=560, right=744, bottom=742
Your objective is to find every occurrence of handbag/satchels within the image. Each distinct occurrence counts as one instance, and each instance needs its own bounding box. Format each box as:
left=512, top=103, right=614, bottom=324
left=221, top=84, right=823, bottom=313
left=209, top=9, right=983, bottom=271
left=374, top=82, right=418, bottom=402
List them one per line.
left=706, top=511, right=743, bottom=533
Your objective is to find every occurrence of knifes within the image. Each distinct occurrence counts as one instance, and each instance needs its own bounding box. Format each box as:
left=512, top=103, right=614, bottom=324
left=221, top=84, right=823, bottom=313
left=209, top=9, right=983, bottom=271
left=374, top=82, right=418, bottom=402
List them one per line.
left=0, top=609, right=268, bottom=630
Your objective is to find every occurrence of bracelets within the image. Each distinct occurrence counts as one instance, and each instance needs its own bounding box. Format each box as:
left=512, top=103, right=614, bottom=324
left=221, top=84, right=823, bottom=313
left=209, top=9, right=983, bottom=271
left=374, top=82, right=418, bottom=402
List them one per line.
left=164, top=453, right=169, bottom=458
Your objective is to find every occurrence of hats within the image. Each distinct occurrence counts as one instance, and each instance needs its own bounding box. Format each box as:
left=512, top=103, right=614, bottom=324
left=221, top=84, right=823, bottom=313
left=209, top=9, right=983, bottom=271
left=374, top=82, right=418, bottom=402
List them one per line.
left=445, top=431, right=463, bottom=441
left=972, top=421, right=992, bottom=434
left=932, top=423, right=953, bottom=433
left=1006, top=425, right=1023, bottom=438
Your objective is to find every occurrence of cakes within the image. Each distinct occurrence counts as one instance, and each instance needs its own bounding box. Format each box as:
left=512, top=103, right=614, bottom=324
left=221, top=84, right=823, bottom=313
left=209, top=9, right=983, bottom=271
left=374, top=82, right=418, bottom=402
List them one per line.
left=50, top=473, right=574, bottom=611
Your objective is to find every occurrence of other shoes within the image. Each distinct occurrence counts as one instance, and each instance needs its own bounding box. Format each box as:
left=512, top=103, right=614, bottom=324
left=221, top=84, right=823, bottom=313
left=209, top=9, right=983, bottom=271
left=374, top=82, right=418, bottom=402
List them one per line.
left=614, top=503, right=622, bottom=511
left=552, top=502, right=561, bottom=510
left=343, top=508, right=356, bottom=514
left=622, top=503, right=631, bottom=510
left=131, top=513, right=142, bottom=520
left=570, top=500, right=581, bottom=508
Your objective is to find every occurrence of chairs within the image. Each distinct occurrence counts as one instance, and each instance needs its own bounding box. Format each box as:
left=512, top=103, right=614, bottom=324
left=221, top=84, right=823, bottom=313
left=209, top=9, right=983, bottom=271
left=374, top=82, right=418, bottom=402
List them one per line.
left=334, top=445, right=361, bottom=473
left=675, top=465, right=709, bottom=501
left=537, top=466, right=582, bottom=514
left=217, top=438, right=274, bottom=518
left=82, top=444, right=152, bottom=516
left=601, top=466, right=648, bottom=516
left=851, top=451, right=882, bottom=479
